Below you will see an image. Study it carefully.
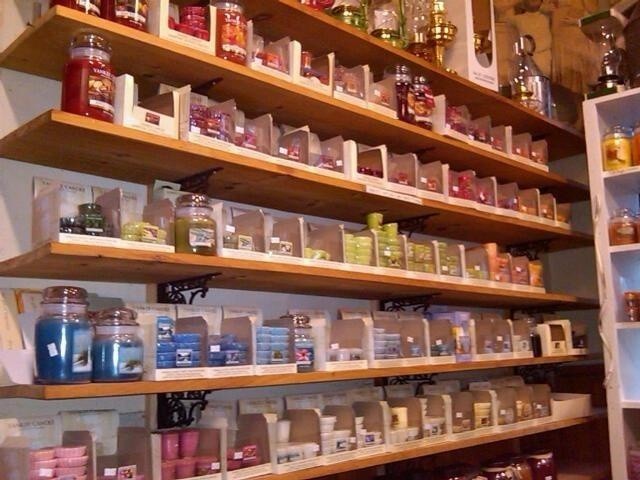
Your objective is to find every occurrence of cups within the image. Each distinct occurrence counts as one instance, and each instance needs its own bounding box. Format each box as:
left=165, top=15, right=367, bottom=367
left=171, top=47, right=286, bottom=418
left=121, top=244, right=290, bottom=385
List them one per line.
left=303, top=232, right=373, bottom=266
left=160, top=428, right=218, bottom=480
left=624, top=290, right=640, bottom=322
left=299, top=51, right=314, bottom=76
left=319, top=413, right=351, bottom=455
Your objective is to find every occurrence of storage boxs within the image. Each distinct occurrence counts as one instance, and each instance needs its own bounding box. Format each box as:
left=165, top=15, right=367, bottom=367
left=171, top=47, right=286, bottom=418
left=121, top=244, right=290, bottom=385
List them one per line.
left=378, top=0, right=498, bottom=92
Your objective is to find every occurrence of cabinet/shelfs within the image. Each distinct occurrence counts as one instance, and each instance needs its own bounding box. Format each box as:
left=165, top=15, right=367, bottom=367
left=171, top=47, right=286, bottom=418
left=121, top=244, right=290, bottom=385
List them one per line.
left=578, top=83, right=640, bottom=479
left=1, top=0, right=610, bottom=479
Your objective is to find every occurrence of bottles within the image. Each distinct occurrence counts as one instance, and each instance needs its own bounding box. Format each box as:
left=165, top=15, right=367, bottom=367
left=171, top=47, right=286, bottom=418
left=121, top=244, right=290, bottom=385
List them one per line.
left=329, top=0, right=401, bottom=38
left=607, top=206, right=640, bottom=245
left=53, top=32, right=117, bottom=127
left=29, top=283, right=142, bottom=382
left=599, top=120, right=640, bottom=172
left=171, top=192, right=220, bottom=257
left=280, top=315, right=315, bottom=372
left=510, top=34, right=553, bottom=117
left=395, top=66, right=436, bottom=131
left=59, top=203, right=110, bottom=237
left=212, top=2, right=248, bottom=67
left=366, top=211, right=461, bottom=276
left=524, top=317, right=543, bottom=357
left=577, top=11, right=629, bottom=99
